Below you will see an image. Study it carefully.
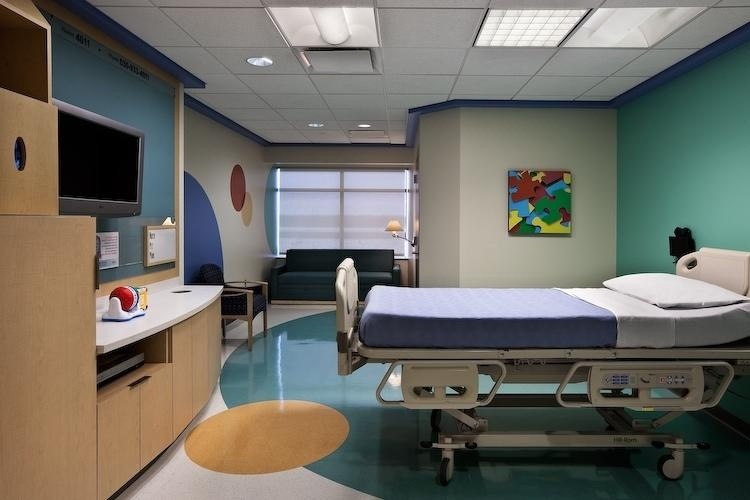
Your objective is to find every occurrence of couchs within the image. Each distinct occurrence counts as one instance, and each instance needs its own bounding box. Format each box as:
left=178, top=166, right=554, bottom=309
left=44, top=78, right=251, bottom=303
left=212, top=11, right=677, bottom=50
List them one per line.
left=268, top=247, right=403, bottom=302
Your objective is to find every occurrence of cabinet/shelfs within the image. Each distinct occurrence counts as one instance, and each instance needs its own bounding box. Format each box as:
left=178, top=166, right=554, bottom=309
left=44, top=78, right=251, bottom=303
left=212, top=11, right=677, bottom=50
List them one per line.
left=0, top=2, right=96, bottom=500
left=96, top=283, right=230, bottom=500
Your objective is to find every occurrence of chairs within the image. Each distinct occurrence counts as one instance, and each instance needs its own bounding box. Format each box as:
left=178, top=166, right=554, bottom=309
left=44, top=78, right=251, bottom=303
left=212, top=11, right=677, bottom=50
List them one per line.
left=191, top=263, right=272, bottom=354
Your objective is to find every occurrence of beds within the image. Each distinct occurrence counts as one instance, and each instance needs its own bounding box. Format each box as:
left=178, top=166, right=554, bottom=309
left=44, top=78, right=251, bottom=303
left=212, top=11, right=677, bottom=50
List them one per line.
left=331, top=241, right=750, bottom=489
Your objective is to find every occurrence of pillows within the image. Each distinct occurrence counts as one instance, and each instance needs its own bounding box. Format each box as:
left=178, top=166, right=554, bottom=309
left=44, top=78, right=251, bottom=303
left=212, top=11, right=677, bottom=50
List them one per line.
left=599, top=271, right=750, bottom=313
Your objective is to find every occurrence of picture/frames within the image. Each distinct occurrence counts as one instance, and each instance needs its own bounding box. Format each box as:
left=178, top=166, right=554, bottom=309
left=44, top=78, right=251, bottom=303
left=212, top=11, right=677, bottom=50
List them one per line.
left=142, top=223, right=179, bottom=268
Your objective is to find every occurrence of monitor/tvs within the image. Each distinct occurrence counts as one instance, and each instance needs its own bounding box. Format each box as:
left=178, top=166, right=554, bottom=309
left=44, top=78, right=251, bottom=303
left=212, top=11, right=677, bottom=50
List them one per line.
left=50, top=97, right=146, bottom=218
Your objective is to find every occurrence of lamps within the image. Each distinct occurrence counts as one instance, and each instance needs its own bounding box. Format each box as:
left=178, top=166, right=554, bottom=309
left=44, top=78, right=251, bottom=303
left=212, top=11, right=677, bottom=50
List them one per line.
left=382, top=217, right=418, bottom=248
